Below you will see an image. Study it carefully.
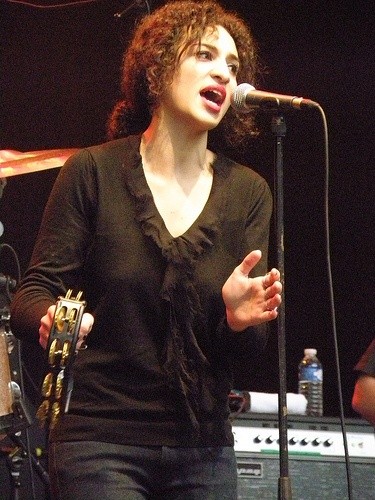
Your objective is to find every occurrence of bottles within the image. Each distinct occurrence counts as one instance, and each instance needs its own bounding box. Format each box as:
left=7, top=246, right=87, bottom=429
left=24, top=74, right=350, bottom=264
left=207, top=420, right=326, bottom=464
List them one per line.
left=298, top=348, right=323, bottom=417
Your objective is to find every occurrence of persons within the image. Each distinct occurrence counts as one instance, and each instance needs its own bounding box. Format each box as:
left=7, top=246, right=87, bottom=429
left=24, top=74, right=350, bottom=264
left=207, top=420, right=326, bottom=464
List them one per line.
left=8, top=0, right=283, bottom=500
left=351, top=339, right=375, bottom=428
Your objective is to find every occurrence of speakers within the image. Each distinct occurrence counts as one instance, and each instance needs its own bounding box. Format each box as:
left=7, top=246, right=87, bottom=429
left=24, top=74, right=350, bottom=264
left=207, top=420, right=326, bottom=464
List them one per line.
left=229, top=413, right=375, bottom=500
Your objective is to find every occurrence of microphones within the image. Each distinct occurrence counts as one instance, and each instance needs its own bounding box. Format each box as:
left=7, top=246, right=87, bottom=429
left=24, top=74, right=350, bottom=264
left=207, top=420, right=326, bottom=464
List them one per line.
left=230, top=83, right=315, bottom=113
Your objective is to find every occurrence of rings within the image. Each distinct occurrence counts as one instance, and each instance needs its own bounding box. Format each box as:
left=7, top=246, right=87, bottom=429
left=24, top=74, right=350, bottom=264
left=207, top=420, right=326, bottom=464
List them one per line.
left=262, top=270, right=279, bottom=311
